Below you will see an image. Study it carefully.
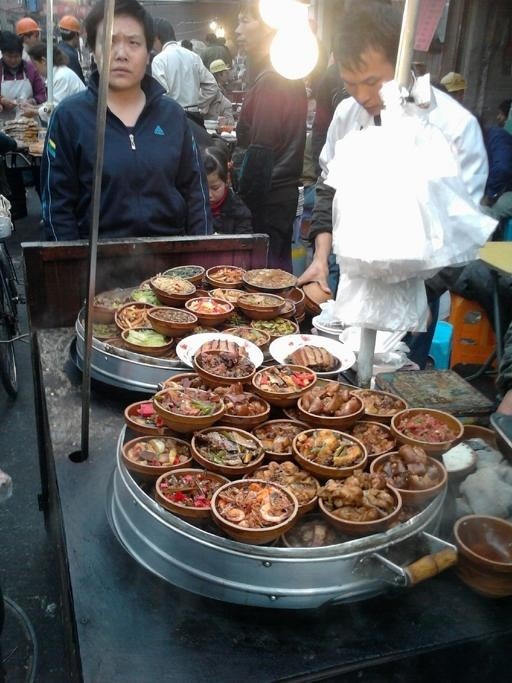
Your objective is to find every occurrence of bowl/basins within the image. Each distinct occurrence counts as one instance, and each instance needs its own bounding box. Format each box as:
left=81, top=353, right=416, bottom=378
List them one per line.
left=237, top=292, right=286, bottom=320
left=139, top=279, right=152, bottom=289
left=239, top=466, right=321, bottom=515
left=120, top=434, right=195, bottom=477
left=350, top=389, right=409, bottom=422
left=205, top=263, right=246, bottom=290
left=297, top=389, right=365, bottom=432
left=163, top=265, right=208, bottom=288
left=113, top=301, right=157, bottom=329
left=251, top=363, right=317, bottom=408
left=293, top=428, right=369, bottom=478
left=250, top=317, right=301, bottom=343
left=217, top=393, right=270, bottom=428
left=150, top=274, right=197, bottom=307
left=120, top=328, right=174, bottom=354
left=454, top=514, right=511, bottom=574
left=318, top=482, right=403, bottom=539
left=370, top=451, right=449, bottom=510
left=152, top=387, right=226, bottom=434
left=209, top=478, right=299, bottom=546
left=131, top=287, right=152, bottom=303
left=251, top=419, right=312, bottom=460
left=390, top=408, right=466, bottom=455
left=194, top=349, right=256, bottom=389
left=122, top=400, right=175, bottom=436
left=220, top=325, right=271, bottom=353
left=155, top=468, right=231, bottom=523
left=238, top=267, right=298, bottom=297
left=190, top=426, right=266, bottom=474
left=180, top=296, right=234, bottom=327
left=146, top=308, right=198, bottom=338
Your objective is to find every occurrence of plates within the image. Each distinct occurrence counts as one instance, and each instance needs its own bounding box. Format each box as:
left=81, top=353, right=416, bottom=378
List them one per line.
left=268, top=334, right=356, bottom=375
left=338, top=326, right=397, bottom=357
left=312, top=314, right=342, bottom=340
left=175, top=332, right=264, bottom=374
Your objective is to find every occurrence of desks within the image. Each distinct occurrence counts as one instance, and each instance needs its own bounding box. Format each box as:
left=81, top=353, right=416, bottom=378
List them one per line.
left=463, top=241, right=511, bottom=384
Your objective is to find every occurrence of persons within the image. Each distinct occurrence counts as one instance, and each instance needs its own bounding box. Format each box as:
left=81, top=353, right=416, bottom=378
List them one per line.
left=194, top=146, right=253, bottom=235
left=231, top=0, right=308, bottom=278
left=2, top=4, right=511, bottom=224
left=492, top=321, right=512, bottom=466
left=42, top=0, right=220, bottom=238
left=294, top=6, right=491, bottom=344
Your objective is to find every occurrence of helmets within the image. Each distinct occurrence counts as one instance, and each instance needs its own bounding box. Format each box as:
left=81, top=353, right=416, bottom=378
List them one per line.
left=58, top=15, right=81, bottom=33
left=209, top=59, right=228, bottom=73
left=16, top=17, right=42, bottom=35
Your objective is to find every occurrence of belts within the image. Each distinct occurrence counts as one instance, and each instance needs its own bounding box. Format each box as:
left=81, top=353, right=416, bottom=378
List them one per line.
left=183, top=107, right=200, bottom=113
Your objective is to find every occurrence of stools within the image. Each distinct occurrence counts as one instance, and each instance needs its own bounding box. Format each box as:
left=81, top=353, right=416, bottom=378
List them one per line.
left=426, top=322, right=456, bottom=367
left=447, top=294, right=500, bottom=367
